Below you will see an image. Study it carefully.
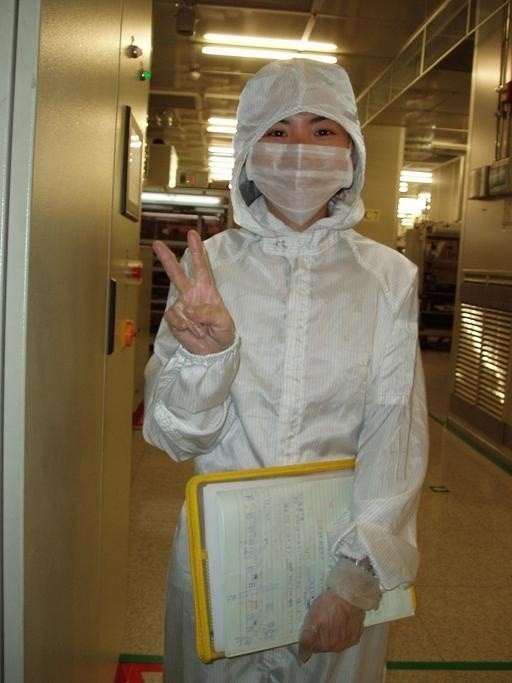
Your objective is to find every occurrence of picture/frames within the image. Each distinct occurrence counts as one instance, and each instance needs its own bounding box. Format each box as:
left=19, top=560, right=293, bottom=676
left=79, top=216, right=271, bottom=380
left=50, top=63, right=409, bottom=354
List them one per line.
left=122, top=104, right=143, bottom=222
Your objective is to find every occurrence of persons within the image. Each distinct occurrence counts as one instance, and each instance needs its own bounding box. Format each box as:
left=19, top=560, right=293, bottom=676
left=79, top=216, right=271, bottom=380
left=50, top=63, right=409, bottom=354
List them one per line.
left=141, top=54, right=433, bottom=682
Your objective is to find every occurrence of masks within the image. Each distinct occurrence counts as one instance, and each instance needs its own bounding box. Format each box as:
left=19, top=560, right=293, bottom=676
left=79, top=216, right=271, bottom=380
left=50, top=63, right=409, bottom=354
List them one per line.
left=246, top=140, right=354, bottom=226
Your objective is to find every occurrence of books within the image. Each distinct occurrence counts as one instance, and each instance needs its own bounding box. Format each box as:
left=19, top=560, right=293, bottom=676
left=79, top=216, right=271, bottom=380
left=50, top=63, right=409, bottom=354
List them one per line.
left=215, top=472, right=417, bottom=657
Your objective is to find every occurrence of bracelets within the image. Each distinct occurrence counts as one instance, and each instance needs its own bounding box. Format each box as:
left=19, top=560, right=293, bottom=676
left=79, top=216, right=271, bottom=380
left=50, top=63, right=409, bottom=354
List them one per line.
left=343, top=553, right=379, bottom=581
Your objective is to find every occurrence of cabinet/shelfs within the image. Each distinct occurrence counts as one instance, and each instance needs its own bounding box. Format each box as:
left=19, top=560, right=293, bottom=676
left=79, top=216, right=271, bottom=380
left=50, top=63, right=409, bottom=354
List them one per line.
left=404, top=227, right=459, bottom=344
